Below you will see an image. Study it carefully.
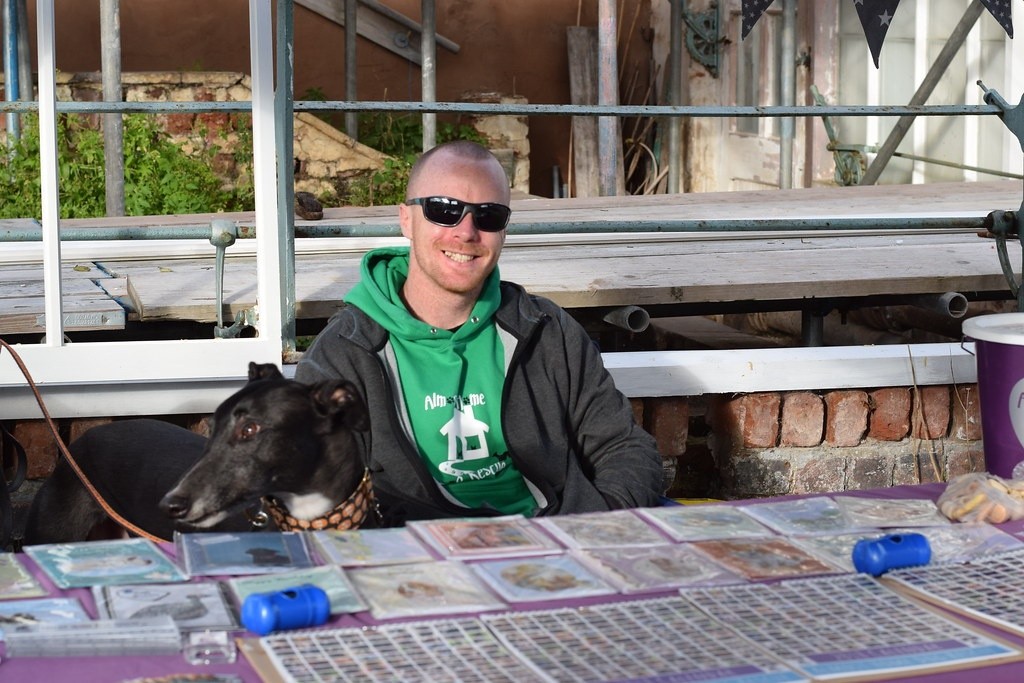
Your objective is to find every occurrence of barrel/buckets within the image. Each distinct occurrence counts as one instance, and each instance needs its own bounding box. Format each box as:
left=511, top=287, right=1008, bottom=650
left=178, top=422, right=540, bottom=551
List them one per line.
left=961, top=312, right=1024, bottom=480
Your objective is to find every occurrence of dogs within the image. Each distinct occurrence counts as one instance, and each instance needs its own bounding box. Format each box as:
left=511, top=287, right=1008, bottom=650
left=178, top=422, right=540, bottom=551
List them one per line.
left=22, top=360, right=372, bottom=546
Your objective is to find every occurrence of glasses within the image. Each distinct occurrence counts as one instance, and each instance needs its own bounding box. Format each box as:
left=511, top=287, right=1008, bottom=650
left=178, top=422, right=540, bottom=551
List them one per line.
left=404, top=197, right=512, bottom=231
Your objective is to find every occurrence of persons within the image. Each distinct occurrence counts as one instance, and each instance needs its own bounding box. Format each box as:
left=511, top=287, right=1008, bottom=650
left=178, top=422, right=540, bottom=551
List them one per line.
left=292, top=140, right=664, bottom=530
